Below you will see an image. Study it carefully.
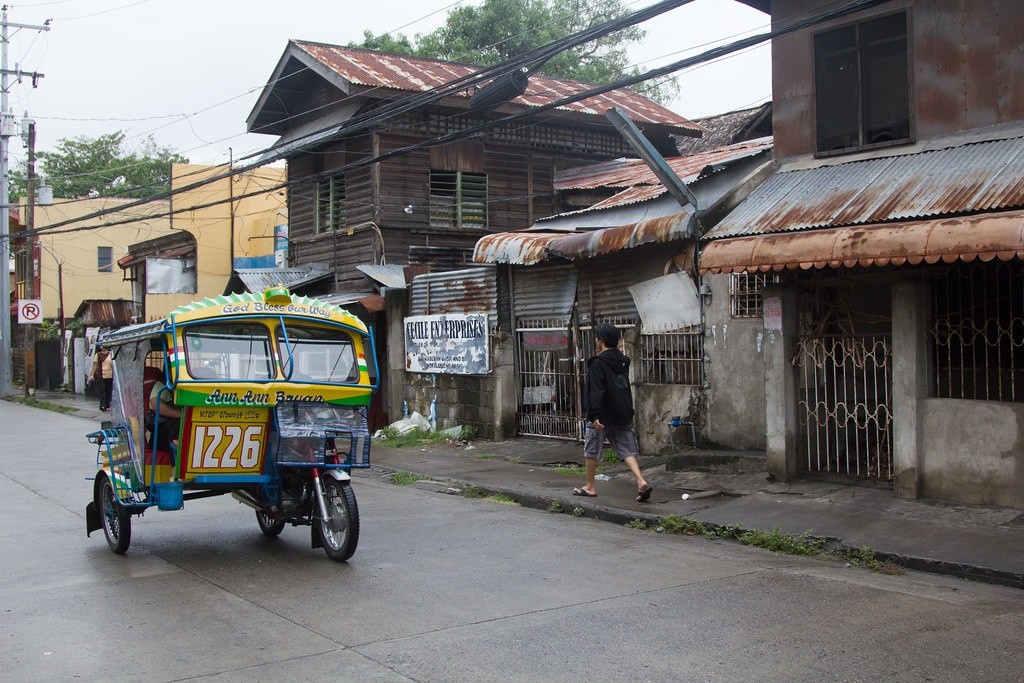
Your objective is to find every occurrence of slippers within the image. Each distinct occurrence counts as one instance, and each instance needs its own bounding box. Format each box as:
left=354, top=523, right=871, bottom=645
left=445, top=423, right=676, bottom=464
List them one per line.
left=572, top=487, right=597, bottom=497
left=637, top=487, right=652, bottom=501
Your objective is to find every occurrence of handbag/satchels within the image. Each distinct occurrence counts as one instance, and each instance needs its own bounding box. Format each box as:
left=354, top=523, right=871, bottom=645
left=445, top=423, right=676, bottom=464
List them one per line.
left=146, top=400, right=180, bottom=424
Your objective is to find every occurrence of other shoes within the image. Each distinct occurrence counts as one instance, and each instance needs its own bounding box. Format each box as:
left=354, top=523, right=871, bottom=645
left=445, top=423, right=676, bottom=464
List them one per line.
left=107, top=407, right=111, bottom=411
left=100, top=407, right=105, bottom=411
left=169, top=477, right=182, bottom=482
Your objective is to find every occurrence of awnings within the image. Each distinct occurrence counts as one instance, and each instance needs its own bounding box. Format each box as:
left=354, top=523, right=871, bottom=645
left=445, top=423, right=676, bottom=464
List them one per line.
left=697, top=209, right=1024, bottom=276
left=472, top=214, right=696, bottom=265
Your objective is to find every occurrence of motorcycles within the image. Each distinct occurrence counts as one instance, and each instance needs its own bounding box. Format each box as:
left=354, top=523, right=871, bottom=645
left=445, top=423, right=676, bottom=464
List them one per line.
left=88, top=286, right=371, bottom=561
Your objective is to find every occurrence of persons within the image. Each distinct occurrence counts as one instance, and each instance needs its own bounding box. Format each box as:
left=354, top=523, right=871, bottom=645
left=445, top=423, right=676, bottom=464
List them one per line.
left=572, top=323, right=653, bottom=501
left=259, top=342, right=313, bottom=381
left=88, top=340, right=113, bottom=411
left=145, top=356, right=184, bottom=482
